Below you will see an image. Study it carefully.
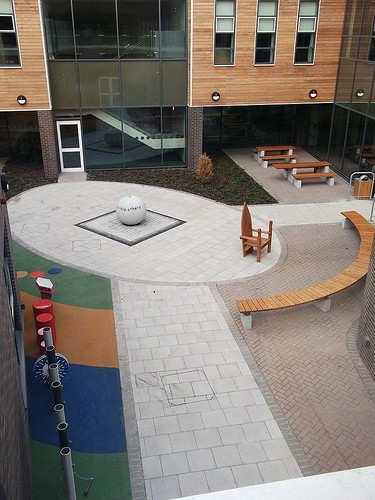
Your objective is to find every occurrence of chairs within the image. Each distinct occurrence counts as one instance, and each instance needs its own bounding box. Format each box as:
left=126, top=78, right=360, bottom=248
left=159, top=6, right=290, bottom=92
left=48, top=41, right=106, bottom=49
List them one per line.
left=240, top=202, right=272, bottom=262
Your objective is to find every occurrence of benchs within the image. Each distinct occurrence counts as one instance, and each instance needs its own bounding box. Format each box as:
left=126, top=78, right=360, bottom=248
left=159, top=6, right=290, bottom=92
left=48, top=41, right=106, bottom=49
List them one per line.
left=253, top=149, right=285, bottom=160
left=292, top=172, right=337, bottom=189
left=359, top=153, right=375, bottom=163
left=368, top=162, right=375, bottom=172
left=237, top=211, right=375, bottom=329
left=259, top=155, right=299, bottom=168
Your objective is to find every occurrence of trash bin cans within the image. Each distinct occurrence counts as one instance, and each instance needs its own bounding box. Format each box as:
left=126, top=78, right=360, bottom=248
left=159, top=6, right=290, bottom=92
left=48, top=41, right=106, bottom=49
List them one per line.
left=32, top=298, right=55, bottom=345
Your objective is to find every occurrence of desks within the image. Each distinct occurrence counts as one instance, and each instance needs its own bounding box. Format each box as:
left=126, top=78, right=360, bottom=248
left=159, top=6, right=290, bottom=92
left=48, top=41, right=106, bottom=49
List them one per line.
left=256, top=146, right=295, bottom=164
left=353, top=145, right=375, bottom=154
left=272, top=161, right=332, bottom=184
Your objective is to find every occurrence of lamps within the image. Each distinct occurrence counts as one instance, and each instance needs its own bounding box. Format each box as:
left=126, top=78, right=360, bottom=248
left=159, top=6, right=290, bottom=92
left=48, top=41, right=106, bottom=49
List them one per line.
left=211, top=92, right=220, bottom=101
left=309, top=89, right=317, bottom=98
left=17, top=95, right=26, bottom=105
